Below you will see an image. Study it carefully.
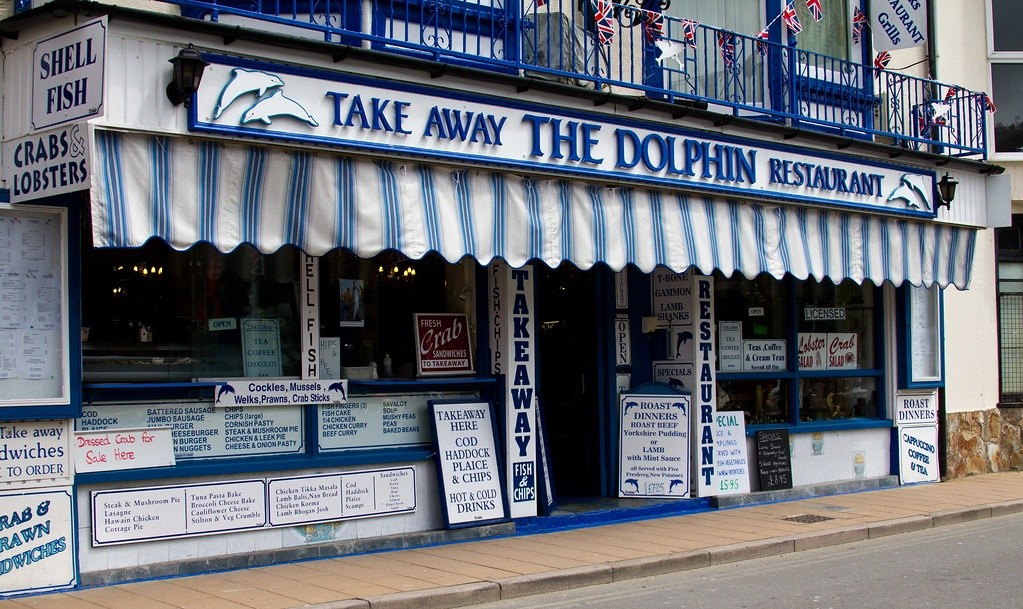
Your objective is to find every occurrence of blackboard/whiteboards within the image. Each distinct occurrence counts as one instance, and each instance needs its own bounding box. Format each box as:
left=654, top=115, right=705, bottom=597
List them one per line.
left=757, top=429, right=793, bottom=490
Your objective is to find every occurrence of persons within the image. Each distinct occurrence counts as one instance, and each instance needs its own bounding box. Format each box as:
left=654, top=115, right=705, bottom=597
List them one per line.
left=342, top=287, right=354, bottom=317
left=852, top=391, right=879, bottom=416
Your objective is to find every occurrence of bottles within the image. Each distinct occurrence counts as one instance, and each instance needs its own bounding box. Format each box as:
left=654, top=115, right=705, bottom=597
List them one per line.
left=372, top=362, right=379, bottom=379
left=382, top=353, right=392, bottom=376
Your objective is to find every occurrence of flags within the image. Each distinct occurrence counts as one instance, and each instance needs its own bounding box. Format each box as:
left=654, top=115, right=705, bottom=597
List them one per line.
left=641, top=9, right=664, bottom=46
left=852, top=6, right=868, bottom=44
left=780, top=3, right=804, bottom=35
left=942, top=83, right=963, bottom=102
left=912, top=104, right=948, bottom=139
left=869, top=0, right=927, bottom=51
left=716, top=27, right=738, bottom=69
left=873, top=50, right=892, bottom=79
left=754, top=26, right=770, bottom=55
left=588, top=0, right=616, bottom=47
left=533, top=0, right=551, bottom=8
left=805, top=0, right=824, bottom=22
left=680, top=18, right=701, bottom=51
left=978, top=92, right=997, bottom=114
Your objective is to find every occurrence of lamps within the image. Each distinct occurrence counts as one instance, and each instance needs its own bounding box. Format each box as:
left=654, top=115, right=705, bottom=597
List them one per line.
left=935, top=171, right=959, bottom=211
left=166, top=42, right=211, bottom=108
